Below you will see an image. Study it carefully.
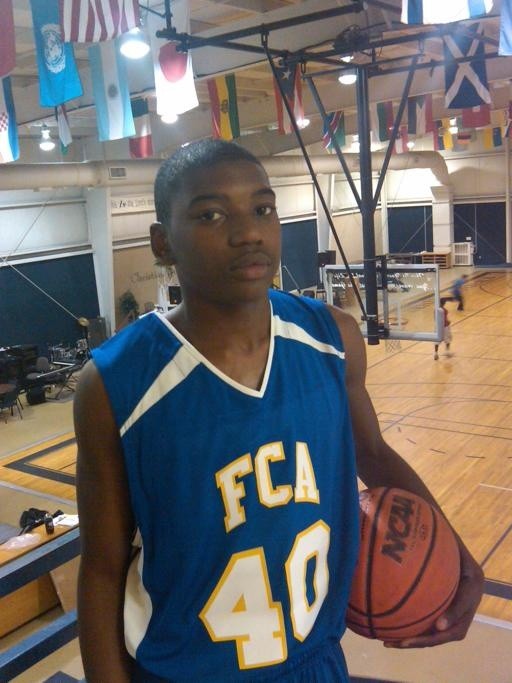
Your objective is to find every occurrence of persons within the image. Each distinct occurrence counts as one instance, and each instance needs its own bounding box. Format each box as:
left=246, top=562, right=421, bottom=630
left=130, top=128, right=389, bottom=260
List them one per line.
left=432, top=296, right=453, bottom=361
left=65, top=131, right=488, bottom=683
left=441, top=272, right=468, bottom=311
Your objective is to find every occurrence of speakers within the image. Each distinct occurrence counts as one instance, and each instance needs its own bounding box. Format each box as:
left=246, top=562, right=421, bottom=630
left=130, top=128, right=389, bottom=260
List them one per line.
left=318, top=252, right=327, bottom=266
left=169, top=286, right=181, bottom=303
left=326, top=250, right=337, bottom=264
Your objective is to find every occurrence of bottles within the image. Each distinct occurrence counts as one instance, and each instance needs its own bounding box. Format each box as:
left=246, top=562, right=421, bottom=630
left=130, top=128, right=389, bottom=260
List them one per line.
left=44, top=511, right=55, bottom=535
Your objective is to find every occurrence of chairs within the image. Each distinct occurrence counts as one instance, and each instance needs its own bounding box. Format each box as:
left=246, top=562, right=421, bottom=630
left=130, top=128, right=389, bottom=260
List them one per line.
left=1, top=355, right=50, bottom=424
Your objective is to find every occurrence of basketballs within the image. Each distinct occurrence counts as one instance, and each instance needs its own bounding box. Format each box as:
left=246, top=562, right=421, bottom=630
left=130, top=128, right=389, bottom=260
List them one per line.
left=346, top=487, right=462, bottom=641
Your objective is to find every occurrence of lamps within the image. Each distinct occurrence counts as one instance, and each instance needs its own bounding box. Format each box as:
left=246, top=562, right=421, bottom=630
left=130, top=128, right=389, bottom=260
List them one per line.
left=295, top=54, right=358, bottom=131
left=116, top=9, right=152, bottom=60
left=38, top=122, right=57, bottom=151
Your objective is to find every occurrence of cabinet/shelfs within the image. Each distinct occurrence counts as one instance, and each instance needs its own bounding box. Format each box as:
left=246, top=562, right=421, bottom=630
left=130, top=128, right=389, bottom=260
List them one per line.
left=383, top=241, right=477, bottom=270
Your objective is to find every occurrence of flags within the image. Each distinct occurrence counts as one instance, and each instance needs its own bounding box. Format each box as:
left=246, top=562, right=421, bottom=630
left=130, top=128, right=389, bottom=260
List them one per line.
left=2, top=75, right=20, bottom=165
left=55, top=104, right=73, bottom=154
left=28, top=1, right=83, bottom=106
left=498, top=2, right=511, bottom=56
left=391, top=125, right=409, bottom=155
left=146, top=1, right=199, bottom=115
left=59, top=0, right=143, bottom=43
left=272, top=64, right=304, bottom=135
left=88, top=37, right=136, bottom=142
left=432, top=109, right=508, bottom=153
left=400, top=1, right=494, bottom=24
left=1, top=1, right=17, bottom=77
left=206, top=73, right=240, bottom=141
left=128, top=97, right=152, bottom=159
left=376, top=100, right=400, bottom=140
left=442, top=22, right=491, bottom=109
left=408, top=93, right=434, bottom=133
left=461, top=83, right=491, bottom=128
left=321, top=111, right=345, bottom=149
